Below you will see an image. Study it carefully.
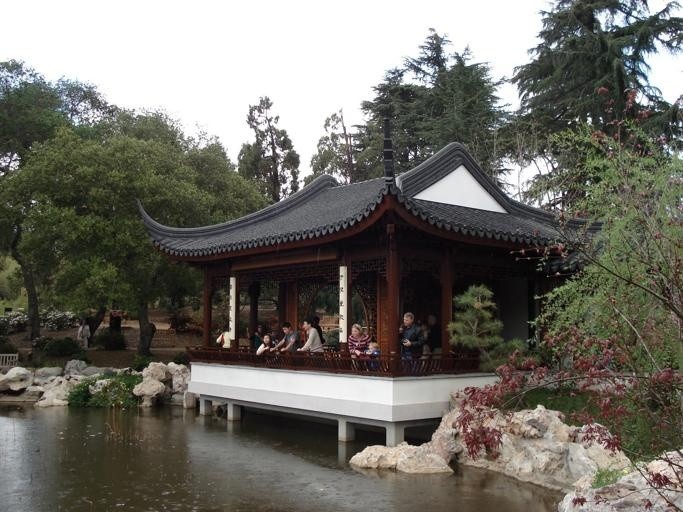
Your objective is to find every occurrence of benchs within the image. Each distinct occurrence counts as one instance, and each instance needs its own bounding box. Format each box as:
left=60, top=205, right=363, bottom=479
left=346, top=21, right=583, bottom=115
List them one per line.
left=0, top=352, right=19, bottom=372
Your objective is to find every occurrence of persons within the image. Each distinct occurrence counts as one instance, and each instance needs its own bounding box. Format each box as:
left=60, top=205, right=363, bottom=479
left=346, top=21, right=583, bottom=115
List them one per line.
left=423, top=313, right=442, bottom=359
left=84, top=310, right=96, bottom=347
left=250, top=316, right=325, bottom=359
left=216, top=325, right=230, bottom=351
left=365, top=342, right=381, bottom=370
left=77, top=318, right=91, bottom=348
left=398, top=312, right=427, bottom=360
left=348, top=323, right=371, bottom=370
